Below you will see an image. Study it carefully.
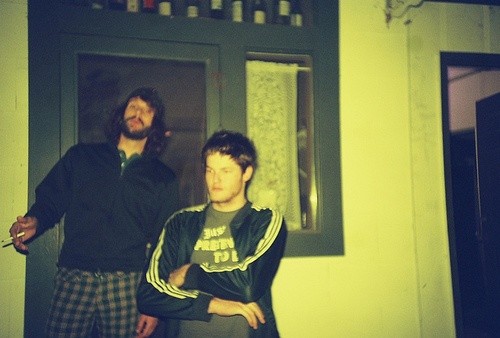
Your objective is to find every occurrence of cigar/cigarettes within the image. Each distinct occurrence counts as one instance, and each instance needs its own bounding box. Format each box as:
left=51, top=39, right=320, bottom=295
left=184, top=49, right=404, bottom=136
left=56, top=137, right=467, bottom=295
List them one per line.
left=1, top=232, right=26, bottom=248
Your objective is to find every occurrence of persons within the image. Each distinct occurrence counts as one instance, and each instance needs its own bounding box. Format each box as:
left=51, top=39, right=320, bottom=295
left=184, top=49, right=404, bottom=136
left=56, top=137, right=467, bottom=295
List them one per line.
left=135, top=130, right=287, bottom=338
left=8, top=86, right=178, bottom=338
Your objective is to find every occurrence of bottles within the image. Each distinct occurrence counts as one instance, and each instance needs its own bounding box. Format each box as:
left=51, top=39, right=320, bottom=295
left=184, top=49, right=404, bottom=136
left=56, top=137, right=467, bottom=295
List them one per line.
left=126, top=0, right=140, bottom=14
left=142, top=0, right=156, bottom=13
left=290, top=0, right=304, bottom=27
left=186, top=0, right=199, bottom=18
left=231, top=0, right=243, bottom=23
left=108, top=0, right=125, bottom=10
left=253, top=0, right=267, bottom=24
left=158, top=0, right=172, bottom=16
left=273, top=0, right=290, bottom=25
left=210, top=0, right=224, bottom=19
left=80, top=0, right=106, bottom=10
